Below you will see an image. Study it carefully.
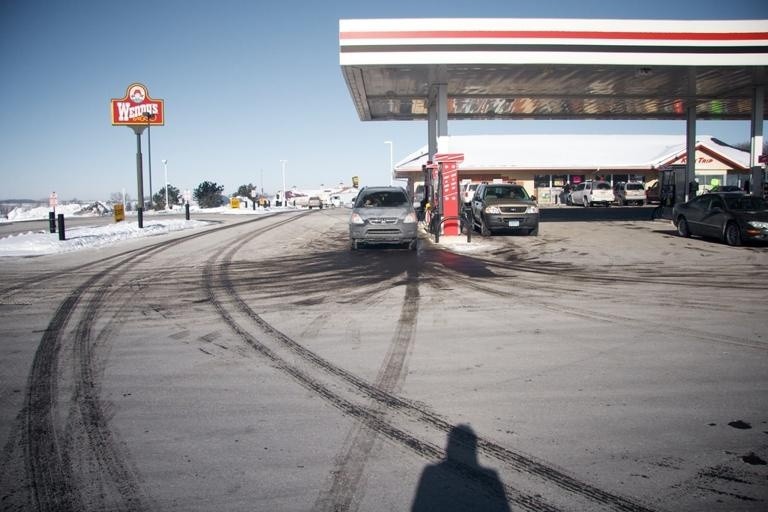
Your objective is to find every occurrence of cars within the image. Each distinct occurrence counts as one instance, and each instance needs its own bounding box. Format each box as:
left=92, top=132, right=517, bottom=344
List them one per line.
left=671, top=186, right=768, bottom=247
left=308, top=197, right=323, bottom=209
left=568, top=182, right=660, bottom=207
left=461, top=182, right=539, bottom=236
left=349, top=187, right=418, bottom=251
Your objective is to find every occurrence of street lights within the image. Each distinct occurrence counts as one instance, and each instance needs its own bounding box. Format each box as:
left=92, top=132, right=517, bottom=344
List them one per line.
left=162, top=160, right=169, bottom=210
left=385, top=141, right=393, bottom=186
left=279, top=160, right=285, bottom=207
